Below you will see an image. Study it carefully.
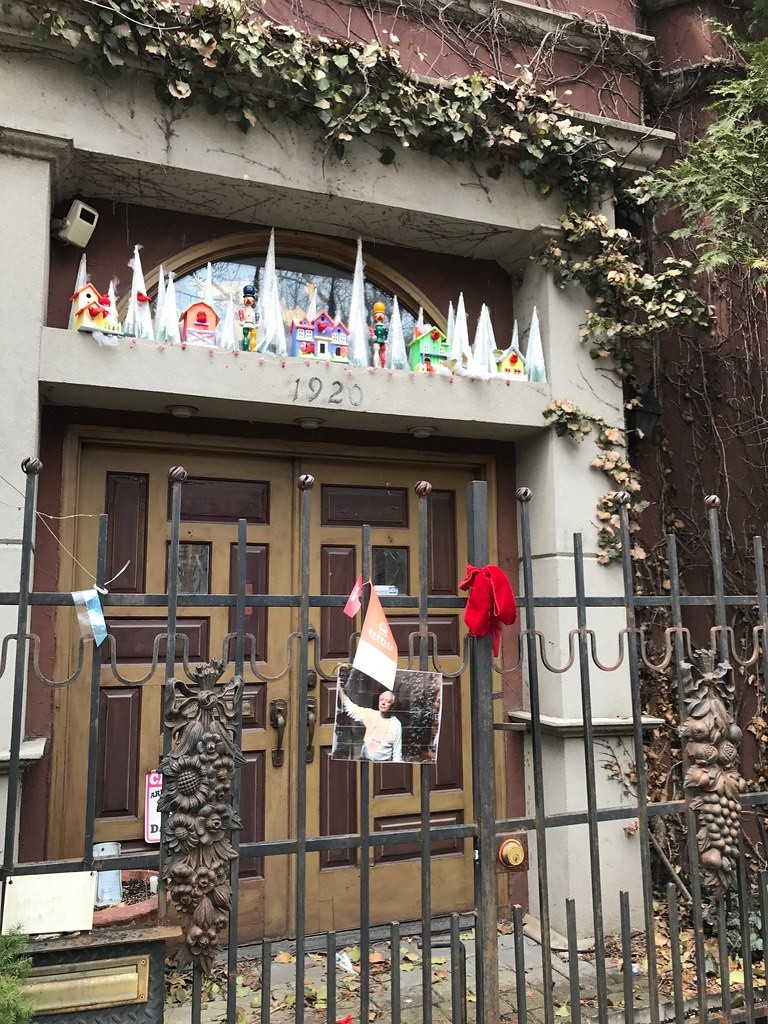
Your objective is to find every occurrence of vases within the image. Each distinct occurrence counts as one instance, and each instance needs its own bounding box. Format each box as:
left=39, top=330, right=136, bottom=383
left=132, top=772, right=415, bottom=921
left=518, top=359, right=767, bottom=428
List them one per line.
left=89, top=870, right=174, bottom=929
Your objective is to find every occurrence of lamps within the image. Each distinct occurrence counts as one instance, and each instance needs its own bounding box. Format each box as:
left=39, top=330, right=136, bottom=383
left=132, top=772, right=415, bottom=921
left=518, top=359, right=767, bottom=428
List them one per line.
left=295, top=416, right=327, bottom=430
left=408, top=425, right=438, bottom=438
left=166, top=405, right=200, bottom=418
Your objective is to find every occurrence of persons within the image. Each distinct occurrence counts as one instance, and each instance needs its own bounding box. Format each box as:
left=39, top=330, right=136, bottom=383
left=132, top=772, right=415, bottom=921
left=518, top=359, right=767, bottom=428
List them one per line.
left=337, top=678, right=403, bottom=763
